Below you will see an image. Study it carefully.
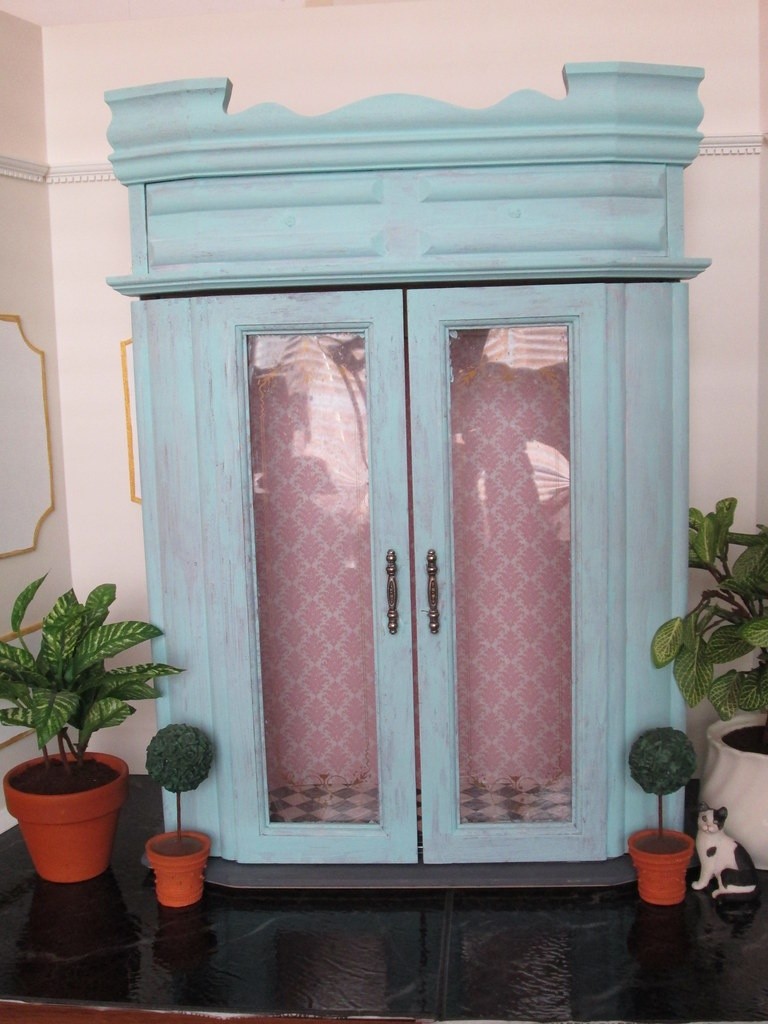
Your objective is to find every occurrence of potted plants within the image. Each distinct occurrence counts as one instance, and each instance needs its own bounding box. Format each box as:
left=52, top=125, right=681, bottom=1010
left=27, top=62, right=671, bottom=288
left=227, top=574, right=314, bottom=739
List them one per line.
left=0, top=568, right=183, bottom=884
left=142, top=725, right=215, bottom=906
left=653, top=493, right=768, bottom=876
left=628, top=725, right=696, bottom=905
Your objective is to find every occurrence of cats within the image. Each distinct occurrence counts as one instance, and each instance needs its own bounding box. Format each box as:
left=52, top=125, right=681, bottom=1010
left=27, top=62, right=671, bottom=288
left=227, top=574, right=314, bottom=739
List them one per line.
left=691, top=800, right=761, bottom=905
